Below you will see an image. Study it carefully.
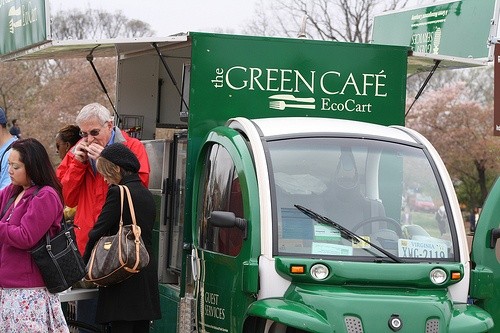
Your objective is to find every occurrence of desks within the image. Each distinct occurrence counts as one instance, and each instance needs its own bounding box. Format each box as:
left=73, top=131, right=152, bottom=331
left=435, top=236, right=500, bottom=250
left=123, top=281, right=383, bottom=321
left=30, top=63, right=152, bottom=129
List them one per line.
left=56, top=288, right=99, bottom=302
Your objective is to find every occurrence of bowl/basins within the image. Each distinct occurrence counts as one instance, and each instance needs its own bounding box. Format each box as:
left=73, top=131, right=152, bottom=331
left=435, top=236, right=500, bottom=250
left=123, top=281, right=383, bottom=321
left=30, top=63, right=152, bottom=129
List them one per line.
left=78, top=281, right=97, bottom=289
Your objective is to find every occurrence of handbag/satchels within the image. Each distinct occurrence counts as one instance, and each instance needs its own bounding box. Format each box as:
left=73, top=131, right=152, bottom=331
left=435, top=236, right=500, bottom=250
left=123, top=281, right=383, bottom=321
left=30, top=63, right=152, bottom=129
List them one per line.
left=84, top=184, right=150, bottom=287
left=28, top=184, right=88, bottom=294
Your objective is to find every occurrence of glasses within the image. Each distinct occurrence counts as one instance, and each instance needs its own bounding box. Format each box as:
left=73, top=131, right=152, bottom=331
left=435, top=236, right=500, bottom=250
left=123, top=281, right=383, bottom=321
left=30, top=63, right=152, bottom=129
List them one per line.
left=79, top=121, right=108, bottom=137
left=57, top=144, right=67, bottom=150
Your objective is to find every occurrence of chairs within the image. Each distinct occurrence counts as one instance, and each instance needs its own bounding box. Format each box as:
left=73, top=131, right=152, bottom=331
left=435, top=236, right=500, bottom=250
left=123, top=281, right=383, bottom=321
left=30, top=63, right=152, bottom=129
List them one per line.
left=341, top=199, right=386, bottom=236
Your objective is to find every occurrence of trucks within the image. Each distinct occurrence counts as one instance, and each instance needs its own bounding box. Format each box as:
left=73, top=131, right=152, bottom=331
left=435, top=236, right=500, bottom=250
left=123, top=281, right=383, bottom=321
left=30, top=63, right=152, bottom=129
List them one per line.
left=0, top=0, right=500, bottom=333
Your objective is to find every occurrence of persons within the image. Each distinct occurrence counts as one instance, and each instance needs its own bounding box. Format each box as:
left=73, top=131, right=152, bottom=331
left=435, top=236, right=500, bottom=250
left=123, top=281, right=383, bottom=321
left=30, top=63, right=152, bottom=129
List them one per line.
left=403, top=207, right=410, bottom=226
left=0, top=138, right=70, bottom=333
left=54, top=102, right=150, bottom=257
left=435, top=205, right=448, bottom=236
left=55, top=124, right=83, bottom=160
left=82, top=143, right=162, bottom=333
left=0, top=107, right=21, bottom=190
left=9, top=119, right=21, bottom=139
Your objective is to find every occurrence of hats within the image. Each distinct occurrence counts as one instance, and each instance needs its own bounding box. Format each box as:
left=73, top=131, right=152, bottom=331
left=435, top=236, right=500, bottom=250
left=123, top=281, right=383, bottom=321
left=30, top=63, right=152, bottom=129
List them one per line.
left=0, top=108, right=7, bottom=124
left=101, top=143, right=141, bottom=173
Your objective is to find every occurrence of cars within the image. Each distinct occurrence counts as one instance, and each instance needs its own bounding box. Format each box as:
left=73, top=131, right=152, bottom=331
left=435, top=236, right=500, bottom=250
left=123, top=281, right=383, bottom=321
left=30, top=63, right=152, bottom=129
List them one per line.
left=408, top=192, right=436, bottom=213
